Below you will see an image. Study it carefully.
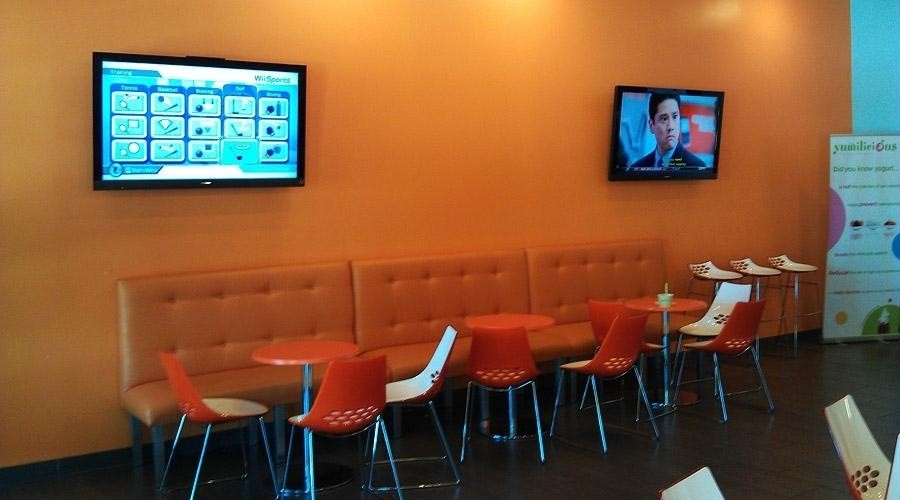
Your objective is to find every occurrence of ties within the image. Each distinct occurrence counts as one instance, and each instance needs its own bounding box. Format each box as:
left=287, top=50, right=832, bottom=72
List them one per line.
left=657, top=158, right=665, bottom=169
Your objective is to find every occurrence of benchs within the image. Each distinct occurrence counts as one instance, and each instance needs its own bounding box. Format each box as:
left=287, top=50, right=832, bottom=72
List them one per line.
left=116, top=260, right=391, bottom=498
left=526, top=236, right=708, bottom=405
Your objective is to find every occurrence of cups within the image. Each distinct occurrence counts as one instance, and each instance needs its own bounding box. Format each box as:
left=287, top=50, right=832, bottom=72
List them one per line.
left=657, top=294, right=674, bottom=309
left=878, top=320, right=890, bottom=334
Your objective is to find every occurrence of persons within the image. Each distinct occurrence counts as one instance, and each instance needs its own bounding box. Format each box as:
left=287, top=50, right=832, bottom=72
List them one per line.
left=630, top=93, right=706, bottom=168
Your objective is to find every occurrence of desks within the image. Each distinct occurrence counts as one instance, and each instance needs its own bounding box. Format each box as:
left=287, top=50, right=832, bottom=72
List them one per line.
left=250, top=341, right=361, bottom=496
left=884, top=434, right=899, bottom=500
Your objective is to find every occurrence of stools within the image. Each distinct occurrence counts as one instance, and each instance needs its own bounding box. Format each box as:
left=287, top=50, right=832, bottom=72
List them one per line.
left=769, top=256, right=820, bottom=356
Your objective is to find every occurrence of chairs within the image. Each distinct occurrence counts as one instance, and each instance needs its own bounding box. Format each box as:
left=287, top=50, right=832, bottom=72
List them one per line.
left=822, top=393, right=893, bottom=500
left=459, top=253, right=774, bottom=465
left=659, top=467, right=724, bottom=500
left=152, top=348, right=275, bottom=499
left=361, top=326, right=464, bottom=491
left=279, top=352, right=405, bottom=499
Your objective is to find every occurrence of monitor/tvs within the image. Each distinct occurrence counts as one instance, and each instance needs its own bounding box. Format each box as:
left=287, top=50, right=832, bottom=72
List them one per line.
left=92, top=51, right=307, bottom=190
left=608, top=85, right=724, bottom=180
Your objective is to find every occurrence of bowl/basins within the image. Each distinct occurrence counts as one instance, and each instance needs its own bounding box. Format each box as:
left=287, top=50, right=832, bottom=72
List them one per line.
left=852, top=225, right=896, bottom=231
